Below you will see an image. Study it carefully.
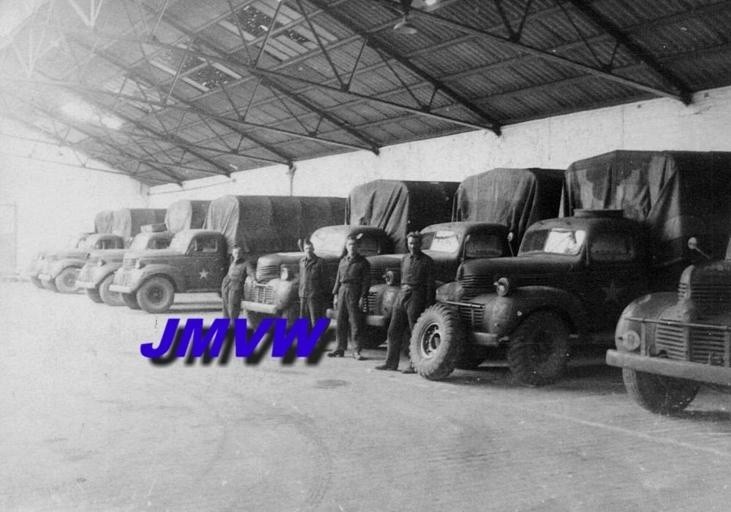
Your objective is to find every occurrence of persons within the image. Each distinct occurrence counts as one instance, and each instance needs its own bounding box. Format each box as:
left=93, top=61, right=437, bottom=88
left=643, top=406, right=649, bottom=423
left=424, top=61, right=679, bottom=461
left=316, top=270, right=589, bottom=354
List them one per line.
left=374, top=232, right=434, bottom=375
left=327, top=235, right=372, bottom=360
left=219, top=244, right=267, bottom=331
left=298, top=240, right=329, bottom=350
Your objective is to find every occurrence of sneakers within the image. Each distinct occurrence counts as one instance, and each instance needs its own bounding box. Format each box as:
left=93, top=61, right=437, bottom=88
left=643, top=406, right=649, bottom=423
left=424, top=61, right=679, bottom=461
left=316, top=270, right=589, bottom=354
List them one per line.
left=353, top=351, right=363, bottom=359
left=375, top=365, right=397, bottom=371
left=403, top=367, right=414, bottom=374
left=327, top=350, right=345, bottom=357
